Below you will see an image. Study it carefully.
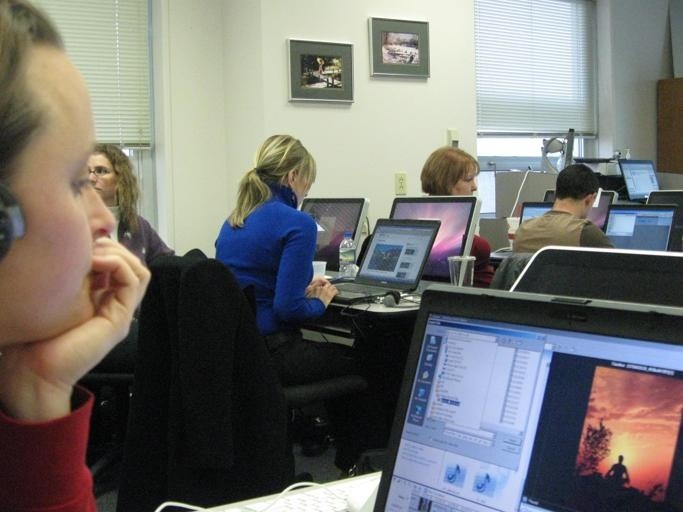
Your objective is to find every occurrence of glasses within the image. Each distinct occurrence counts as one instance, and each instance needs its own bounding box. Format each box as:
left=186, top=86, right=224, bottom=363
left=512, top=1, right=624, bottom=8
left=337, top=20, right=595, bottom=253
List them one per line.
left=85, top=166, right=118, bottom=176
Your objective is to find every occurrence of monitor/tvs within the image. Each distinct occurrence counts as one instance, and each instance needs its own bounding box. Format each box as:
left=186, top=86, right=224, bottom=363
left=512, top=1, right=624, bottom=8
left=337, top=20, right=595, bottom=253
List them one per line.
left=508, top=245, right=683, bottom=306
left=389, top=195, right=482, bottom=289
left=601, top=204, right=679, bottom=250
left=301, top=197, right=370, bottom=277
left=645, top=189, right=683, bottom=252
left=373, top=289, right=683, bottom=512
left=542, top=188, right=556, bottom=203
left=586, top=189, right=619, bottom=233
left=618, top=158, right=661, bottom=203
left=519, top=201, right=553, bottom=223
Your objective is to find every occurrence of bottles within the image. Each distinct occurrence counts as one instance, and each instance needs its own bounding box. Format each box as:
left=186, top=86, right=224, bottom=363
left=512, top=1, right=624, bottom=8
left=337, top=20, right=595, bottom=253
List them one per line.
left=338, top=231, right=356, bottom=278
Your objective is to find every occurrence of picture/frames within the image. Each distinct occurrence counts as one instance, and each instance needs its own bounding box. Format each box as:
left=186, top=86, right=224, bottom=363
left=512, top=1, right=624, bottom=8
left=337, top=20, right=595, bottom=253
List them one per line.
left=286, top=38, right=354, bottom=103
left=369, top=17, right=430, bottom=78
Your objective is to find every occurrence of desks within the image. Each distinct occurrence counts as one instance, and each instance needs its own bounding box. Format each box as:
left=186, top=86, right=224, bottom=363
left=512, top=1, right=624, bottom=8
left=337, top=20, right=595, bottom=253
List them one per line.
left=195, top=470, right=384, bottom=512
left=300, top=270, right=421, bottom=347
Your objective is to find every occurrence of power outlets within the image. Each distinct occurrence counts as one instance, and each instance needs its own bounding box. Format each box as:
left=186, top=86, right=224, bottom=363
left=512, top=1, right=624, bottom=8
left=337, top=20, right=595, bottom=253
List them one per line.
left=395, top=173, right=407, bottom=195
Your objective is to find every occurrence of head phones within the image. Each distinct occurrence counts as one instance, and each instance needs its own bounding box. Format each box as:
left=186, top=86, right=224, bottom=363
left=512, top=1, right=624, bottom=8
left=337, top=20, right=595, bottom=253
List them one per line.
left=383, top=289, right=420, bottom=308
left=0, top=183, right=26, bottom=260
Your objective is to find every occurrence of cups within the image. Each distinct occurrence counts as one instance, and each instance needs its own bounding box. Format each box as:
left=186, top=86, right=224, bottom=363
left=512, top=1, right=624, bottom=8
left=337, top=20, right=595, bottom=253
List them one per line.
left=507, top=218, right=520, bottom=247
left=312, top=261, right=327, bottom=280
left=448, top=256, right=476, bottom=287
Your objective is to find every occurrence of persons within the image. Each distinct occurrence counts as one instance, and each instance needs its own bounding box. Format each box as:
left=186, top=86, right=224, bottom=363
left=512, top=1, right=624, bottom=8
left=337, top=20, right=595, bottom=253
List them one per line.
left=0, top=0, right=145, bottom=511
left=214, top=134, right=378, bottom=480
left=420, top=145, right=492, bottom=290
left=87, top=141, right=175, bottom=354
left=512, top=164, right=618, bottom=268
left=605, top=454, right=633, bottom=493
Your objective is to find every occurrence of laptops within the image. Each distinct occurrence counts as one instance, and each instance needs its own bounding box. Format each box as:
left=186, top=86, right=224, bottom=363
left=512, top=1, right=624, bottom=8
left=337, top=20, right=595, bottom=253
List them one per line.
left=328, top=218, right=441, bottom=305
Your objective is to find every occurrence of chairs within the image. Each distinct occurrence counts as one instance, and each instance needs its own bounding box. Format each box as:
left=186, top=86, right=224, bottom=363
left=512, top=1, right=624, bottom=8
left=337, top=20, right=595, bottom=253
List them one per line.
left=79, top=318, right=140, bottom=477
left=150, top=254, right=368, bottom=484
